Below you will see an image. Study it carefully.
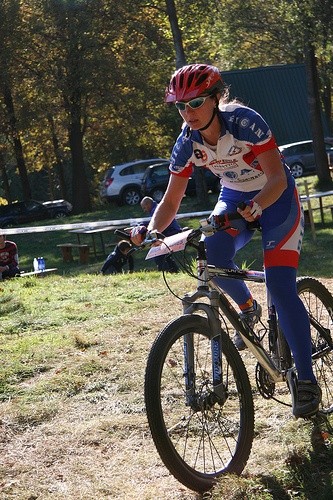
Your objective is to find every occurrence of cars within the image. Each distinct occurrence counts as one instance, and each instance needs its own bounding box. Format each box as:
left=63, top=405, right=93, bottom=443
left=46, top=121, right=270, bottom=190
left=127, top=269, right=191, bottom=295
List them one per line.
left=0, top=199, right=73, bottom=228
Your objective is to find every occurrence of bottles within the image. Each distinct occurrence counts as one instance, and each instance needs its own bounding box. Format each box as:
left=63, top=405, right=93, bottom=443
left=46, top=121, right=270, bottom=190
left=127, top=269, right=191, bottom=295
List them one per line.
left=37, top=257, right=45, bottom=270
left=32, top=258, right=38, bottom=272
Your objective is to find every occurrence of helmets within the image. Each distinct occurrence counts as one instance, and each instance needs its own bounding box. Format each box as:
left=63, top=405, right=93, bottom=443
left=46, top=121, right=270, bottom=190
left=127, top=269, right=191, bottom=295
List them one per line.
left=163, top=64, right=224, bottom=104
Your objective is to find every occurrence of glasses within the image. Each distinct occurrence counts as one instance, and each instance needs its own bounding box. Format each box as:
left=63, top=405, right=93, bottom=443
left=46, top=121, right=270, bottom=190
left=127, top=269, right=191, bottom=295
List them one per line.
left=175, top=94, right=214, bottom=112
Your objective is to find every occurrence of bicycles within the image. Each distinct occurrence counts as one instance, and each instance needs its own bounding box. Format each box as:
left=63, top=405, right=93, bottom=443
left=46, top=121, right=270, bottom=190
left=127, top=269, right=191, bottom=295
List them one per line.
left=112, top=227, right=333, bottom=493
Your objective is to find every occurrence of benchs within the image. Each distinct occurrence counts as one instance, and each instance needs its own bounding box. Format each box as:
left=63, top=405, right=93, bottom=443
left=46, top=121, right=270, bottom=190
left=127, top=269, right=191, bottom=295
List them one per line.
left=304, top=203, right=333, bottom=227
left=56, top=242, right=91, bottom=263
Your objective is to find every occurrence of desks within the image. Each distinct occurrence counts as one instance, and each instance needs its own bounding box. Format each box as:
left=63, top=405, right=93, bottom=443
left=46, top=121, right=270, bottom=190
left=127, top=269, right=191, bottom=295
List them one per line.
left=67, top=223, right=127, bottom=261
left=299, top=190, right=333, bottom=231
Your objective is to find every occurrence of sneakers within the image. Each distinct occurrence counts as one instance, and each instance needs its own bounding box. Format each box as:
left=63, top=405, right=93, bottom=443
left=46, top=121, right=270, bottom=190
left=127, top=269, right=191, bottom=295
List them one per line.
left=234, top=300, right=262, bottom=350
left=293, top=380, right=322, bottom=418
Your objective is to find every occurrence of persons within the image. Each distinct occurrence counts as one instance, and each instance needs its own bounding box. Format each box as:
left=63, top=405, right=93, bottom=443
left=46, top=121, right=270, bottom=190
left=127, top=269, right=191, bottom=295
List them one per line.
left=101, top=240, right=131, bottom=275
left=130, top=64, right=322, bottom=417
left=124, top=196, right=181, bottom=273
left=0, top=234, right=19, bottom=280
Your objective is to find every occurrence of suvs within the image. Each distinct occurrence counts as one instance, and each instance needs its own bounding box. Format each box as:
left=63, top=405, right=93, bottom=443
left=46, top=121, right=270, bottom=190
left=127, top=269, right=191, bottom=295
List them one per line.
left=276, top=140, right=333, bottom=179
left=100, top=159, right=169, bottom=207
left=141, top=161, right=222, bottom=204
